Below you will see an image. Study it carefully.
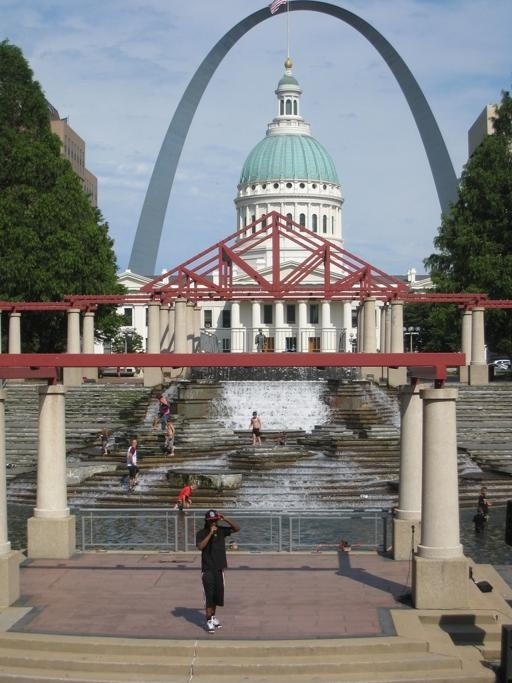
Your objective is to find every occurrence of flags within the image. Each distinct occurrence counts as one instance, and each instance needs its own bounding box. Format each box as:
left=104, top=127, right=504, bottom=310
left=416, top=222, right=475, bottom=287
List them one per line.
left=268, top=0, right=288, bottom=15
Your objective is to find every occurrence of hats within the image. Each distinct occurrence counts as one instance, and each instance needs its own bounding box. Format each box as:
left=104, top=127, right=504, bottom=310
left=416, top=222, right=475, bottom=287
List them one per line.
left=204, top=509, right=221, bottom=522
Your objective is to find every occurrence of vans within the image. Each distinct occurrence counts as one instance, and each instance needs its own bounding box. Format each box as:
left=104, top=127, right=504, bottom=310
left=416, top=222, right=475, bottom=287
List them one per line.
left=494, top=359, right=511, bottom=367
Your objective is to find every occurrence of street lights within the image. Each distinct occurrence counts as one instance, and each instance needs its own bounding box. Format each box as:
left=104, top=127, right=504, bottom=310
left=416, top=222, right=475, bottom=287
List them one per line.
left=404, top=326, right=420, bottom=353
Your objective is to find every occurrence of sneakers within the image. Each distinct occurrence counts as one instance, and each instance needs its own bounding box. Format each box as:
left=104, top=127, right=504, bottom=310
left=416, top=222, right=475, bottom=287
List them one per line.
left=206, top=615, right=224, bottom=634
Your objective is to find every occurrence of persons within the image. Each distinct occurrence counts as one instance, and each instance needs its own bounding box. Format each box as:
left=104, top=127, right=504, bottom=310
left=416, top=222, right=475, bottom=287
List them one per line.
left=340, top=537, right=352, bottom=551
left=228, top=540, right=239, bottom=550
left=195, top=509, right=241, bottom=635
left=165, top=420, right=176, bottom=457
left=158, top=395, right=170, bottom=434
left=246, top=411, right=263, bottom=446
left=478, top=486, right=493, bottom=523
left=126, top=439, right=141, bottom=494
left=471, top=506, right=489, bottom=536
left=101, top=427, right=110, bottom=455
left=177, top=481, right=198, bottom=515
left=255, top=328, right=268, bottom=352
